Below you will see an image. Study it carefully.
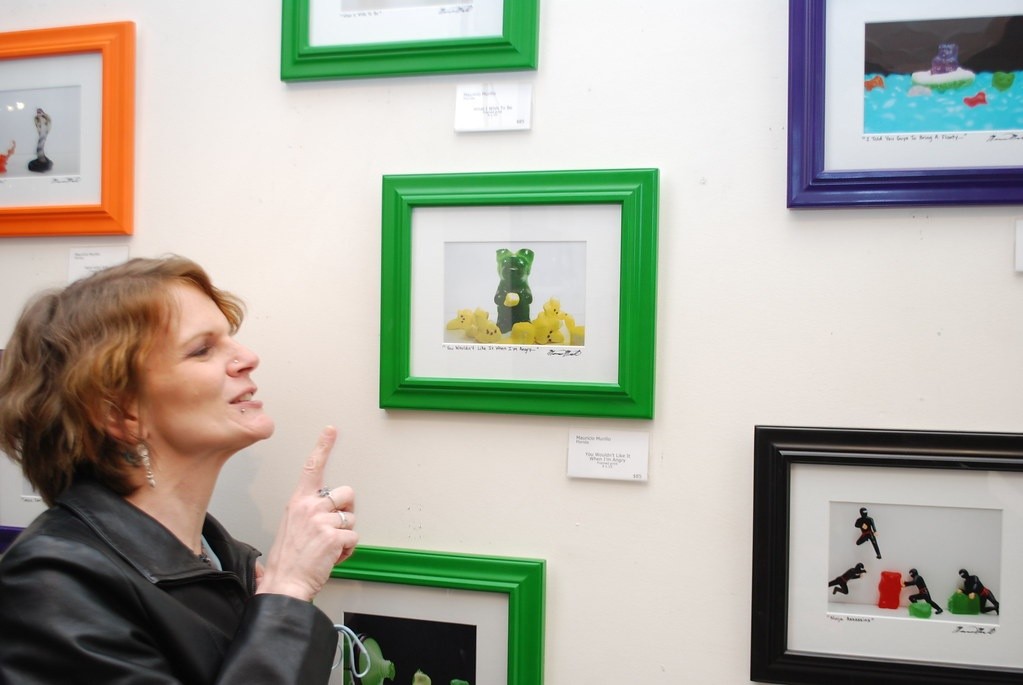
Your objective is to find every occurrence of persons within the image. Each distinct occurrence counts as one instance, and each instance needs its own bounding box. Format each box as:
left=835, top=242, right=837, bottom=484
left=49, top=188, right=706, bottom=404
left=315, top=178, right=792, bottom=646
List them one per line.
left=0, top=254, right=359, bottom=685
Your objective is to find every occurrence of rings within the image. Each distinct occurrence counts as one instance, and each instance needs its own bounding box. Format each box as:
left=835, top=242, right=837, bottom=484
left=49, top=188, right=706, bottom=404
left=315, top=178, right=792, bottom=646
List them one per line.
left=319, top=484, right=338, bottom=509
left=337, top=511, right=347, bottom=529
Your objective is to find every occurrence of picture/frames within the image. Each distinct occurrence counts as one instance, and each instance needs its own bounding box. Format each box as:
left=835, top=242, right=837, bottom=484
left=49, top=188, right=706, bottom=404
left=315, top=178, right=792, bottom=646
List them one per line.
left=310, top=545, right=547, bottom=685
left=0, top=18, right=135, bottom=238
left=375, top=165, right=660, bottom=421
left=748, top=422, right=1023, bottom=685
left=282, top=0, right=542, bottom=84
left=785, top=0, right=1023, bottom=208
